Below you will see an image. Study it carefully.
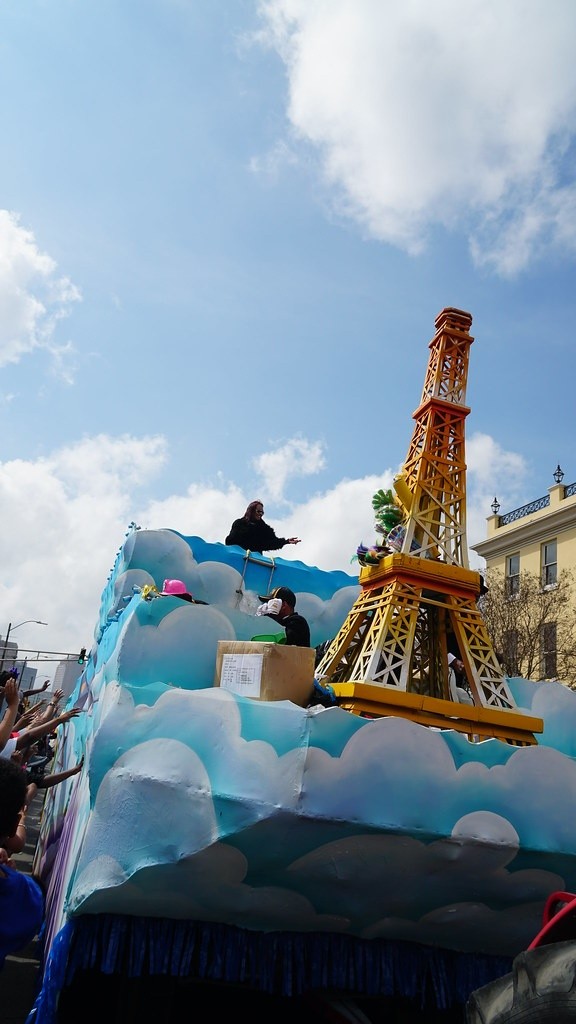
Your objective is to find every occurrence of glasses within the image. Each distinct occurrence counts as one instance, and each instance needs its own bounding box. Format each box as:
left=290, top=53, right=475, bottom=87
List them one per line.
left=255, top=510, right=264, bottom=515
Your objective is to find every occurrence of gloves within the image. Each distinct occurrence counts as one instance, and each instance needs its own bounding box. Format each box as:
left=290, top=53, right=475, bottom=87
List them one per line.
left=255, top=603, right=267, bottom=616
left=268, top=598, right=282, bottom=615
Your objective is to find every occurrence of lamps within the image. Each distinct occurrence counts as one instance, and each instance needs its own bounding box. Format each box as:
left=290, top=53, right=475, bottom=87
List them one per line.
left=553, top=465, right=565, bottom=483
left=491, top=497, right=501, bottom=515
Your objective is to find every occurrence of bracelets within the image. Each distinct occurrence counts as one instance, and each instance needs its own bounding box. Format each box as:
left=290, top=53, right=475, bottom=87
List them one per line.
left=46, top=702, right=55, bottom=707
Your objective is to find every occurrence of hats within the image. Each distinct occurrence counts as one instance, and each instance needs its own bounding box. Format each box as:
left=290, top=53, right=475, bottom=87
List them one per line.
left=158, top=580, right=186, bottom=596
left=258, top=586, right=296, bottom=607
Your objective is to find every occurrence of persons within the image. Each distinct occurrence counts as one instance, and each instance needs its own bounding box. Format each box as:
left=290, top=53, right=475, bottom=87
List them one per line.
left=448, top=652, right=475, bottom=706
left=258, top=587, right=311, bottom=648
left=225, top=501, right=301, bottom=555
left=0, top=668, right=85, bottom=962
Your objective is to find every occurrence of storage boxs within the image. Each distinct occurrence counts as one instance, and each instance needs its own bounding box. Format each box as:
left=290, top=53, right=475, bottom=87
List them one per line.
left=214, top=640, right=317, bottom=708
